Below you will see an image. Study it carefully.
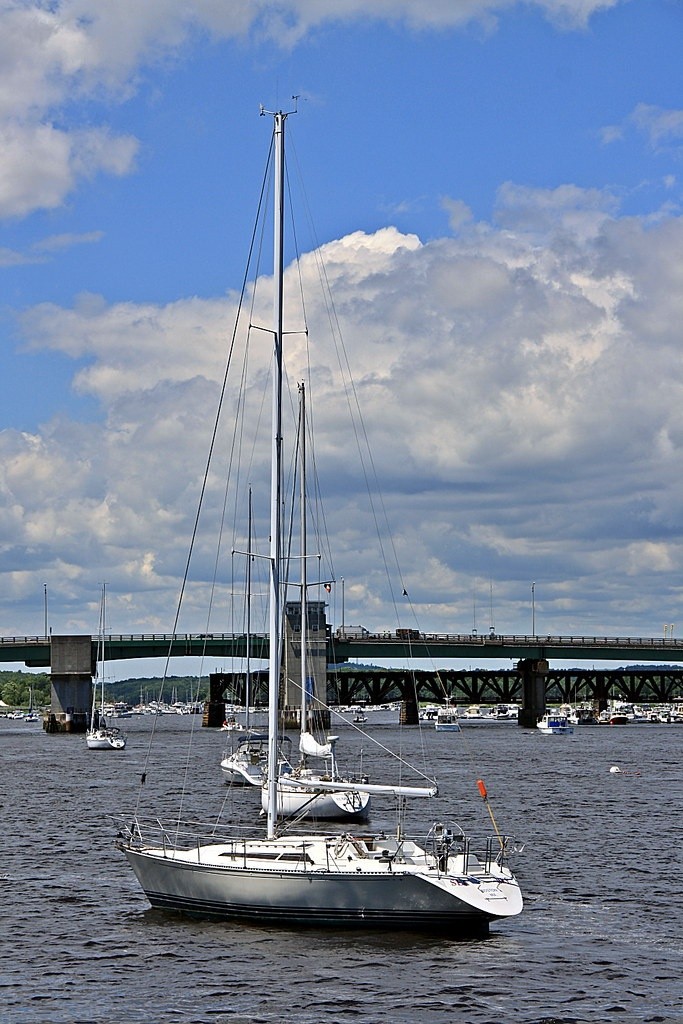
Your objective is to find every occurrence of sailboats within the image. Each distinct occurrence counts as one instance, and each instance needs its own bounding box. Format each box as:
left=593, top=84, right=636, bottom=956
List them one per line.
left=220, top=482, right=299, bottom=788
left=92, top=685, right=206, bottom=718
left=86, top=579, right=129, bottom=750
left=99, top=94, right=525, bottom=925
left=199, top=377, right=440, bottom=820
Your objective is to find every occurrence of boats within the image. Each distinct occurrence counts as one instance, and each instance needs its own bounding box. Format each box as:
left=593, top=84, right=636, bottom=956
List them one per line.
left=327, top=698, right=683, bottom=724
left=435, top=694, right=461, bottom=732
left=0, top=711, right=44, bottom=722
left=299, top=731, right=340, bottom=760
left=535, top=712, right=575, bottom=735
